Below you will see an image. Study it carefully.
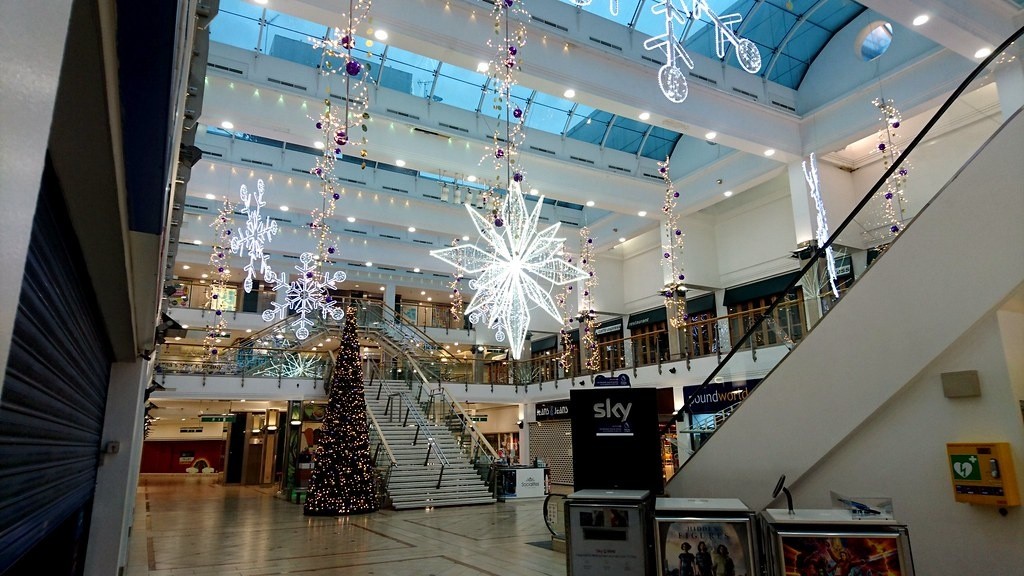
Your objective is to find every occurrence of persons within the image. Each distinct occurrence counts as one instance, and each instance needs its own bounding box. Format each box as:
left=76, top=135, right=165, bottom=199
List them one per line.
left=678, top=541, right=735, bottom=576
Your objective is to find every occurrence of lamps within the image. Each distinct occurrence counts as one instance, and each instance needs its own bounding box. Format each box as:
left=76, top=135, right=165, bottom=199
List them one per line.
left=200, top=0, right=913, bottom=371
